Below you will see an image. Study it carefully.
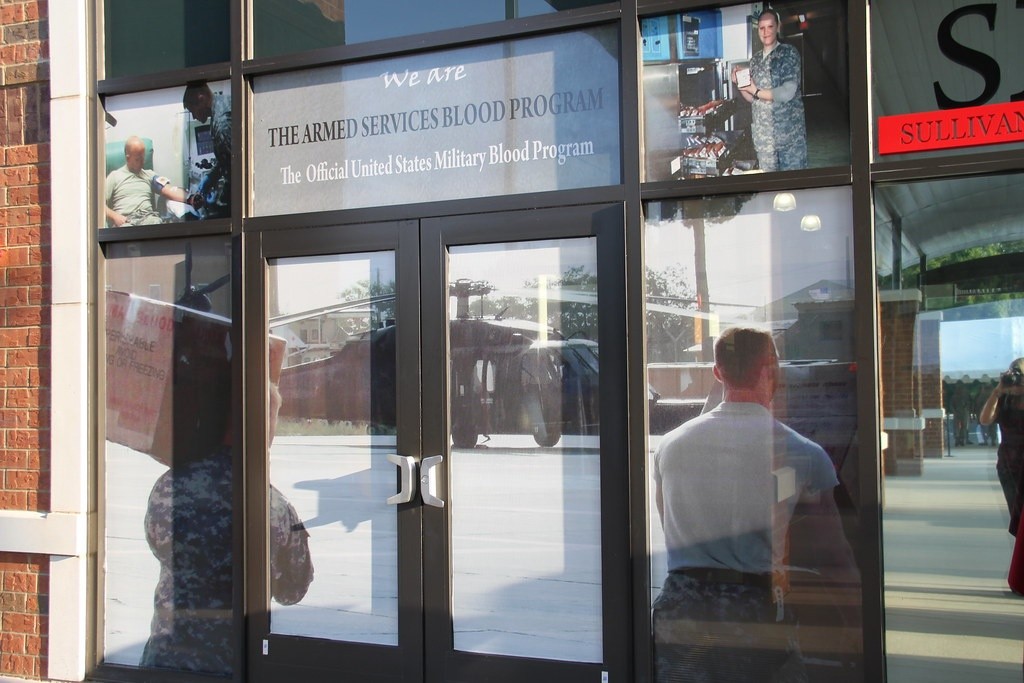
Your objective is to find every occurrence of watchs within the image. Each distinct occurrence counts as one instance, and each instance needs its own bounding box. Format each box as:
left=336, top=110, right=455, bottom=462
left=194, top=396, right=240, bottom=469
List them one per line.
left=186, top=194, right=194, bottom=203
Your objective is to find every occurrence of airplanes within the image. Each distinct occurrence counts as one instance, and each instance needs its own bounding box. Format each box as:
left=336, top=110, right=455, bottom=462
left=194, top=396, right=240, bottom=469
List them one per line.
left=173, top=242, right=230, bottom=313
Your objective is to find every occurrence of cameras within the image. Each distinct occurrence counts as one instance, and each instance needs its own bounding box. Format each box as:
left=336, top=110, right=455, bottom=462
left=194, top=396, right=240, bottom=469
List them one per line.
left=1002, top=368, right=1024, bottom=386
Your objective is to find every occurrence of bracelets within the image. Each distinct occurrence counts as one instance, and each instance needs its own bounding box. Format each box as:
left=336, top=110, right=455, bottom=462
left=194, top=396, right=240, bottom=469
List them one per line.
left=753, top=89, right=760, bottom=99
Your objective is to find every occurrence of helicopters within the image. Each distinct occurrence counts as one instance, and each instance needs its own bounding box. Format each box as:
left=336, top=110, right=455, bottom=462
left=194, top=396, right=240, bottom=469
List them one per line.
left=269, top=276, right=764, bottom=451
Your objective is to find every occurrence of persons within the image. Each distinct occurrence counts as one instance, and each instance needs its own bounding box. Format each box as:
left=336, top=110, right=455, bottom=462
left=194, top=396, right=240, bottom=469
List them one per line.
left=654, top=326, right=862, bottom=683
left=134, top=384, right=315, bottom=674
left=980, top=358, right=1024, bottom=597
left=183, top=82, right=232, bottom=219
left=949, top=381, right=974, bottom=446
left=732, top=8, right=808, bottom=173
left=105, top=135, right=205, bottom=229
left=977, top=383, right=998, bottom=447
